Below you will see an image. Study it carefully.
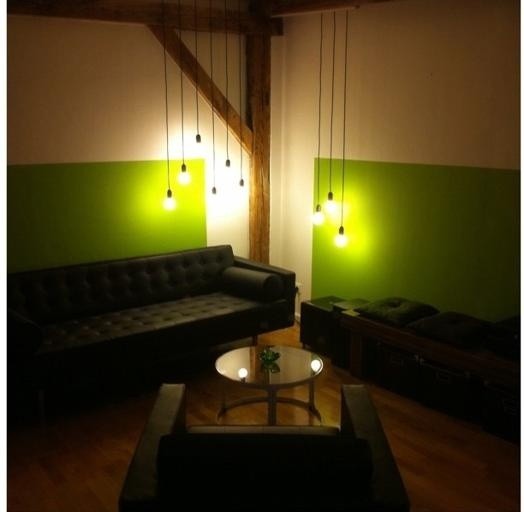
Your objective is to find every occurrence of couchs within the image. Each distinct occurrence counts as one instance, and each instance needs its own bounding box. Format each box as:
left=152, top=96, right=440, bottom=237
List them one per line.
left=6, top=244, right=298, bottom=376
left=116, top=382, right=412, bottom=512
left=298, top=293, right=520, bottom=417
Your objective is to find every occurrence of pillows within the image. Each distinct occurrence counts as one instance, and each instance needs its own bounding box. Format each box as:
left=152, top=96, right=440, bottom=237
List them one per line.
left=407, top=309, right=494, bottom=350
left=353, top=296, right=438, bottom=326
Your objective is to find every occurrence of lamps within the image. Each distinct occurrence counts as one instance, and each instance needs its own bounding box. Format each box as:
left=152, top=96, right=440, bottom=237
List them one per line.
left=309, top=359, right=321, bottom=374
left=313, top=11, right=349, bottom=247
left=237, top=368, right=248, bottom=383
left=159, top=3, right=251, bottom=209
